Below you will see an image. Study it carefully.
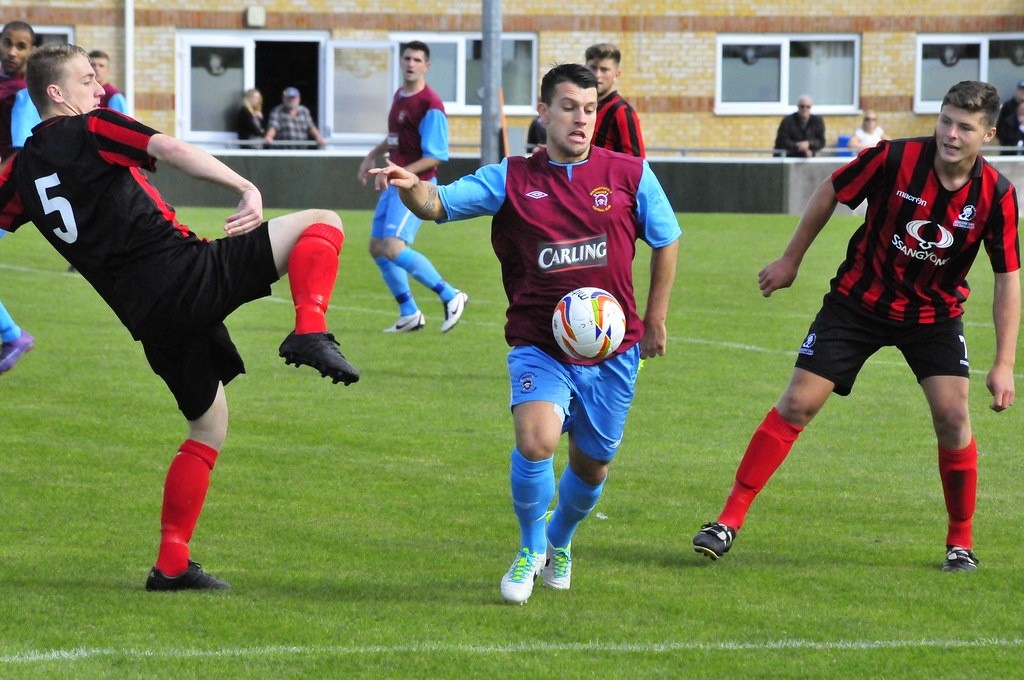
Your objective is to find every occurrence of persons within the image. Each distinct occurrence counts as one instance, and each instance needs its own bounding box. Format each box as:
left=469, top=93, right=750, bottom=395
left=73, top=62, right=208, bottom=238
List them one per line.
left=692, top=79, right=1021, bottom=573
left=847, top=109, right=890, bottom=149
left=263, top=87, right=327, bottom=149
left=236, top=88, right=264, bottom=148
left=85, top=49, right=126, bottom=116
left=367, top=63, right=684, bottom=604
left=0, top=43, right=361, bottom=592
left=357, top=41, right=471, bottom=332
left=582, top=43, right=647, bottom=159
left=773, top=94, right=826, bottom=157
left=0, top=20, right=37, bottom=373
left=993, top=79, right=1024, bottom=155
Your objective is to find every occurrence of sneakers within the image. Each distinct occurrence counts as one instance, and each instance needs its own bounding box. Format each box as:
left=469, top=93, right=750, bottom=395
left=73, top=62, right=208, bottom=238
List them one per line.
left=545, top=511, right=572, bottom=589
left=500, top=544, right=547, bottom=603
left=145, top=561, right=233, bottom=593
left=943, top=545, right=978, bottom=573
left=279, top=328, right=361, bottom=387
left=694, top=520, right=736, bottom=559
left=0, top=328, right=34, bottom=372
left=382, top=310, right=425, bottom=333
left=439, top=289, right=469, bottom=333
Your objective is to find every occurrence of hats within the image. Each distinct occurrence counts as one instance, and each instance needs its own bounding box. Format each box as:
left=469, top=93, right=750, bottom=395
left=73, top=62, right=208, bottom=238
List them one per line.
left=283, top=87, right=299, bottom=98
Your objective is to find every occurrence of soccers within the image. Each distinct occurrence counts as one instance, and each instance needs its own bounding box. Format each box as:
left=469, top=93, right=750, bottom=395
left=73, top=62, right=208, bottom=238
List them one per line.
left=551, top=286, right=628, bottom=362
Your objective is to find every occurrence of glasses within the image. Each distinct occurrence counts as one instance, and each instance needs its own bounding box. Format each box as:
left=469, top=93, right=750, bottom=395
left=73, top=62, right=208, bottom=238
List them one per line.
left=865, top=117, right=877, bottom=121
left=798, top=104, right=811, bottom=109
